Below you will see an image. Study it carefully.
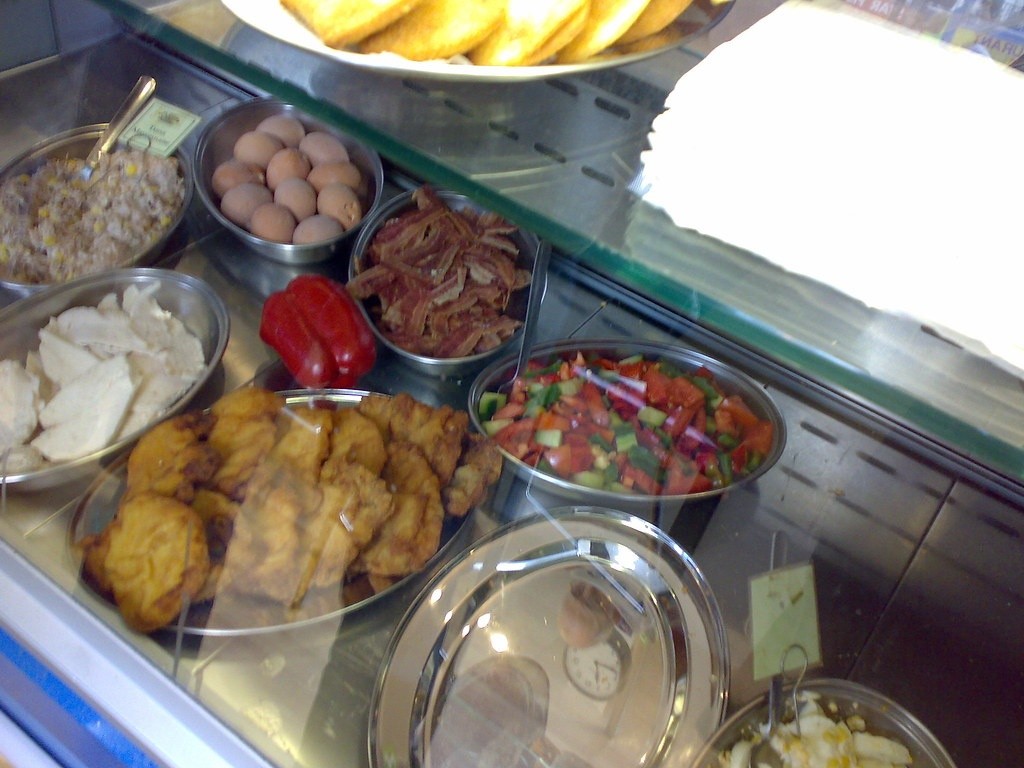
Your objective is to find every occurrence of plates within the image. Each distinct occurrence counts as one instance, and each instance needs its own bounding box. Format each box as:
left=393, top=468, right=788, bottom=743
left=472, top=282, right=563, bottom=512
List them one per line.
left=218, top=1, right=744, bottom=80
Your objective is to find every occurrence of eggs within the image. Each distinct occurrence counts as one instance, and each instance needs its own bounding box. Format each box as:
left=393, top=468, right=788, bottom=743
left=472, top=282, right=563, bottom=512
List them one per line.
left=212, top=114, right=362, bottom=246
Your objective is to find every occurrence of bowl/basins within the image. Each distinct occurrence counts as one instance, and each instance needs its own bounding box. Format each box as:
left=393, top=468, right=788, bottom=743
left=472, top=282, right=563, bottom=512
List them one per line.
left=69, top=385, right=483, bottom=636
left=347, top=185, right=547, bottom=377
left=193, top=97, right=383, bottom=263
left=690, top=680, right=955, bottom=768
left=0, top=122, right=195, bottom=292
left=0, top=264, right=231, bottom=492
left=469, top=338, right=787, bottom=508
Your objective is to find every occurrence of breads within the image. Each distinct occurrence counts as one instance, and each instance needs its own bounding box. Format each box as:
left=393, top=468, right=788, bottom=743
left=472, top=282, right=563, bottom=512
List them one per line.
left=279, top=0, right=696, bottom=71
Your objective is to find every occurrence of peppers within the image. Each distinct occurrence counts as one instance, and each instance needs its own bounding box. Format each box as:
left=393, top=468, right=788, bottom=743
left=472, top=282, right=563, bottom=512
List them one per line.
left=486, top=357, right=774, bottom=496
left=258, top=273, right=378, bottom=391
